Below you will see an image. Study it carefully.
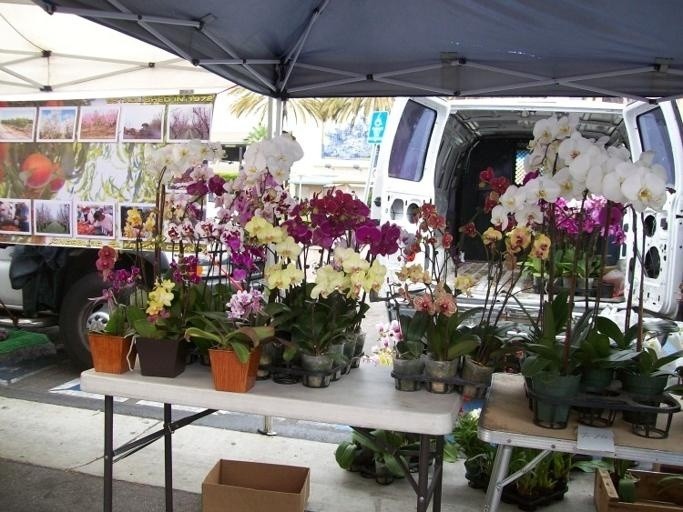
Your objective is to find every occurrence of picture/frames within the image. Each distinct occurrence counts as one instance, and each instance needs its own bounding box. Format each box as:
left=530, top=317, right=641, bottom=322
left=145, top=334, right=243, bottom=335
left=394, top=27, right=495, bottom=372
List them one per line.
left=0, top=198, right=205, bottom=244
left=0, top=102, right=212, bottom=144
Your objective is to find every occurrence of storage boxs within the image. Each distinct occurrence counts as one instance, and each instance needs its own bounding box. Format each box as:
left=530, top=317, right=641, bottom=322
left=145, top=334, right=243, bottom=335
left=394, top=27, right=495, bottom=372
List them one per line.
left=199, top=459, right=311, bottom=510
left=590, top=465, right=683, bottom=512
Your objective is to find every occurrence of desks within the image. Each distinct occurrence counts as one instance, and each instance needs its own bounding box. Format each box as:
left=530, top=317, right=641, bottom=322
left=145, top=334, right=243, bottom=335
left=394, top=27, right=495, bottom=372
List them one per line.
left=79, top=354, right=464, bottom=512
left=476, top=369, right=682, bottom=512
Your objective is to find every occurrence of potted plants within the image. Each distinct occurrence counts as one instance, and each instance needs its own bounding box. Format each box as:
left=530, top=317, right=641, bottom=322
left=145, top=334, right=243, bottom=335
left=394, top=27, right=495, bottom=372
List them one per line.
left=201, top=131, right=401, bottom=393
left=87, top=143, right=201, bottom=379
left=455, top=412, right=642, bottom=511
left=524, top=111, right=682, bottom=440
left=369, top=117, right=551, bottom=398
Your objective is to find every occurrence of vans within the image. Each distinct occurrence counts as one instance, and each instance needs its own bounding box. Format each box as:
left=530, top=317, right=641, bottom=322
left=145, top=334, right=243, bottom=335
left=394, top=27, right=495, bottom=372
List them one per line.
left=357, top=90, right=680, bottom=343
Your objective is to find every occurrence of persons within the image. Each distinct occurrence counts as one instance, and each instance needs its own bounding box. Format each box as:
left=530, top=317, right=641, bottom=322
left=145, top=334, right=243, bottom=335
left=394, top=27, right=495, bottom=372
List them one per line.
left=78, top=206, right=113, bottom=236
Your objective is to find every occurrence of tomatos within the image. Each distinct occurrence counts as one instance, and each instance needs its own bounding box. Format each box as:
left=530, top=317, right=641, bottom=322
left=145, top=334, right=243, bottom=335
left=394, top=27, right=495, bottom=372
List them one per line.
left=0, top=142, right=66, bottom=193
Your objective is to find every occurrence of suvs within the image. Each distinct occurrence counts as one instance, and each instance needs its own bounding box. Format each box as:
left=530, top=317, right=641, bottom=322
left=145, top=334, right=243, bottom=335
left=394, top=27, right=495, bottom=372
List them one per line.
left=0, top=219, right=272, bottom=374
